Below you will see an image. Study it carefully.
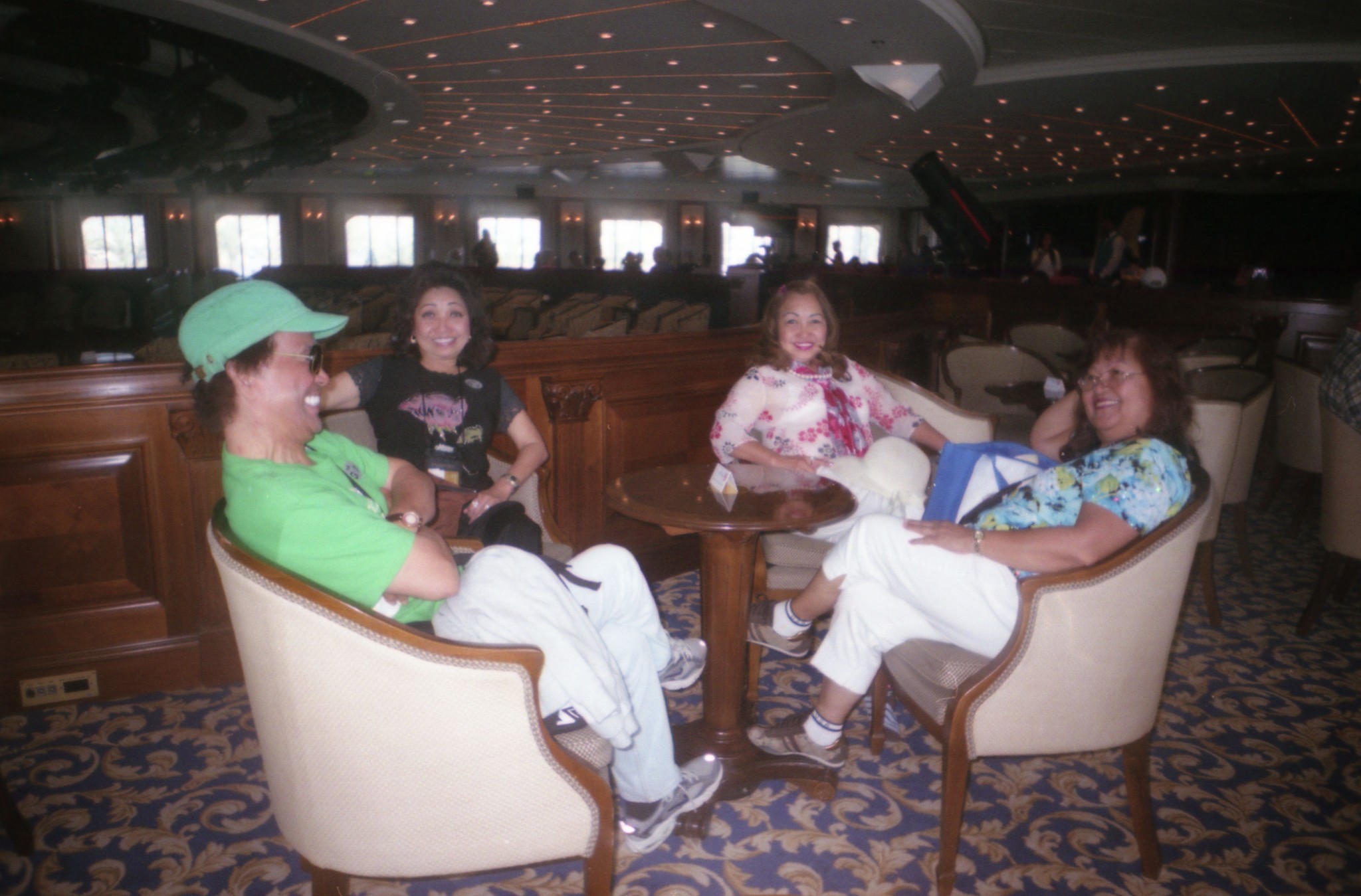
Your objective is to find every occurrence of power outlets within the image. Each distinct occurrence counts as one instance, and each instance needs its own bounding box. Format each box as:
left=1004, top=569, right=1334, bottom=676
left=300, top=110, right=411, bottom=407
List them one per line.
left=20, top=671, right=98, bottom=707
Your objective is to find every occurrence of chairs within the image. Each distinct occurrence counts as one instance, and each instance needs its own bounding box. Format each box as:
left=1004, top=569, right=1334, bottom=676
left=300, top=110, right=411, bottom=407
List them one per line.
left=0, top=290, right=1361, bottom=896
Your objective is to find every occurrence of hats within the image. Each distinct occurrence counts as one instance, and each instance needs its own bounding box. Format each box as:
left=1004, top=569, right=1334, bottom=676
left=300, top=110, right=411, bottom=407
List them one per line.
left=178, top=279, right=348, bottom=384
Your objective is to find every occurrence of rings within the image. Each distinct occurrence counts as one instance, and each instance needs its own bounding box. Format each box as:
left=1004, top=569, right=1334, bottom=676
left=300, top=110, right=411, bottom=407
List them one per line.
left=484, top=505, right=488, bottom=510
left=473, top=500, right=478, bottom=507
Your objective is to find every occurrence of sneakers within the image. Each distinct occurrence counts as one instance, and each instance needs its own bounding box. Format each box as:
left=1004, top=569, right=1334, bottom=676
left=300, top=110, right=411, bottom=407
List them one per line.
left=745, top=592, right=812, bottom=657
left=658, top=638, right=707, bottom=690
left=616, top=754, right=724, bottom=852
left=746, top=708, right=848, bottom=767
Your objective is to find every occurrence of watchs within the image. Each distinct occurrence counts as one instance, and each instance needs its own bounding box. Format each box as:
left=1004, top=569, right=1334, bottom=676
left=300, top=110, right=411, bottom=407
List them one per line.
left=501, top=473, right=520, bottom=488
left=973, top=530, right=984, bottom=553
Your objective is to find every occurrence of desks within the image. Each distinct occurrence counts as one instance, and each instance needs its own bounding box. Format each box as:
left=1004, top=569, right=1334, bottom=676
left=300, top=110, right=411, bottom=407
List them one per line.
left=983, top=377, right=1077, bottom=417
left=604, top=461, right=858, bottom=842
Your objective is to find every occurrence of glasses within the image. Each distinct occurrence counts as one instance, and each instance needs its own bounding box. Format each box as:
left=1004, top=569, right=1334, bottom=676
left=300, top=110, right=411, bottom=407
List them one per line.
left=268, top=346, right=322, bottom=374
left=1079, top=368, right=1147, bottom=394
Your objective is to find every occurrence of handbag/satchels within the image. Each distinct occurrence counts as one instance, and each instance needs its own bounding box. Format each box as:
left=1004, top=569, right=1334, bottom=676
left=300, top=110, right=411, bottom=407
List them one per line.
left=457, top=502, right=601, bottom=616
left=425, top=471, right=477, bottom=536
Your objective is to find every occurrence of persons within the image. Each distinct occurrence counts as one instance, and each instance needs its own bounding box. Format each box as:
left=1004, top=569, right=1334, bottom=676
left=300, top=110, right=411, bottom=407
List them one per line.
left=469, top=230, right=497, bottom=266
left=916, top=233, right=934, bottom=269
left=177, top=278, right=723, bottom=854
left=832, top=241, right=843, bottom=264
left=534, top=248, right=720, bottom=275
left=320, top=263, right=548, bottom=560
left=1027, top=230, right=1061, bottom=274
left=709, top=280, right=954, bottom=543
left=743, top=314, right=1204, bottom=768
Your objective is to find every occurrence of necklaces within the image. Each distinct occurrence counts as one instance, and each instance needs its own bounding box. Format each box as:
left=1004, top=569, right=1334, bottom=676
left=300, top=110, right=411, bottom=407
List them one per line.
left=786, top=366, right=833, bottom=379
